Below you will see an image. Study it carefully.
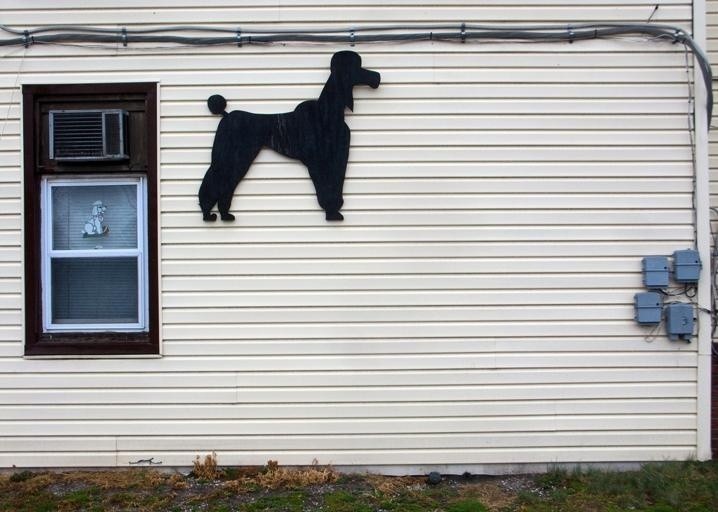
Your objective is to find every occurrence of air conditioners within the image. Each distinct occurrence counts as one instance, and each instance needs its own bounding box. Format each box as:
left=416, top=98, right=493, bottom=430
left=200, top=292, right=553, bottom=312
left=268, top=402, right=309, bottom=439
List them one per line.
left=48, top=109, right=130, bottom=161
